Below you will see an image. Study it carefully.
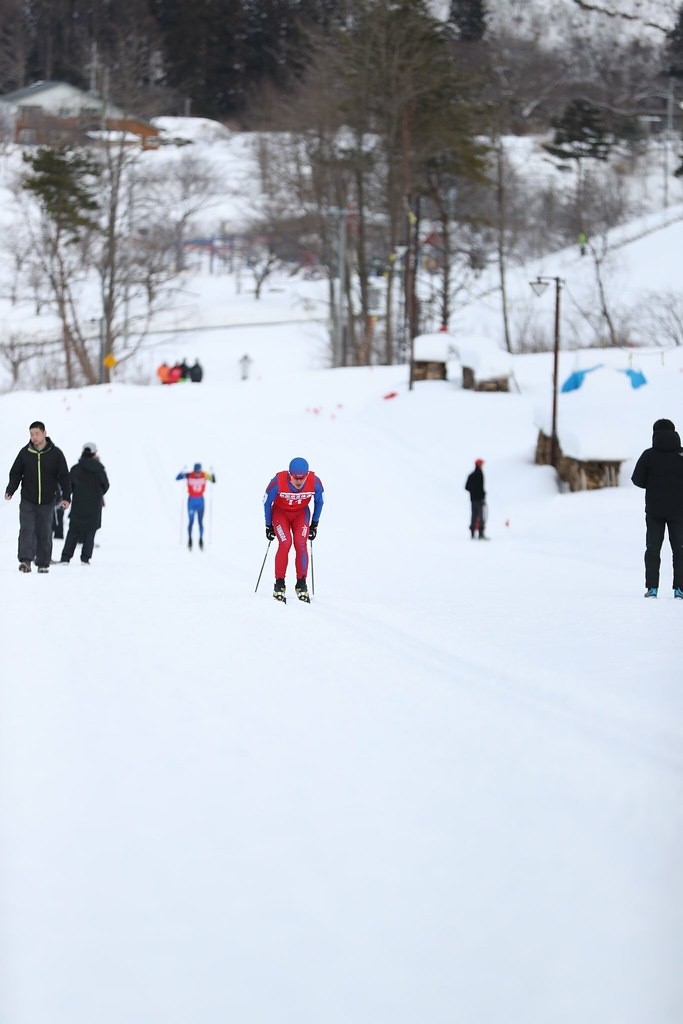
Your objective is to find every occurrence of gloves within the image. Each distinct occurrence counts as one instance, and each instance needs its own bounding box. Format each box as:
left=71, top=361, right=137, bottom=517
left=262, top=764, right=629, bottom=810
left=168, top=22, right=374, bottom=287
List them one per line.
left=266, top=523, right=275, bottom=541
left=308, top=521, right=318, bottom=540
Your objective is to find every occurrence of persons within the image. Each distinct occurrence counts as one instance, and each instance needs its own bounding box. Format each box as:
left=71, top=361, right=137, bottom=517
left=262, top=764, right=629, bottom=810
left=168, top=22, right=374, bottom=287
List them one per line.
left=157, top=358, right=202, bottom=385
left=263, top=457, right=324, bottom=603
left=52, top=442, right=110, bottom=563
left=239, top=355, right=251, bottom=380
left=176, top=463, right=216, bottom=551
left=5, top=421, right=72, bottom=573
left=466, top=459, right=489, bottom=540
left=631, top=418, right=683, bottom=600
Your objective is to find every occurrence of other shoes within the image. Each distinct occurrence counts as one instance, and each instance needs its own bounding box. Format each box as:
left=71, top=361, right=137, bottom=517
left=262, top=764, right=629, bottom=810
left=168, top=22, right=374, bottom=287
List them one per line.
left=60, top=557, right=70, bottom=563
left=81, top=555, right=89, bottom=563
left=273, top=584, right=285, bottom=600
left=295, top=584, right=309, bottom=602
left=644, top=587, right=657, bottom=597
left=19, top=559, right=31, bottom=572
left=674, top=587, right=683, bottom=598
left=38, top=565, right=48, bottom=572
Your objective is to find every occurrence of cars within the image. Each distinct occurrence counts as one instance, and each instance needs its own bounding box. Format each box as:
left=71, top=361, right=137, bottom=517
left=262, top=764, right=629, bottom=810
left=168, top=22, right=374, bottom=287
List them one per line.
left=74, top=115, right=231, bottom=149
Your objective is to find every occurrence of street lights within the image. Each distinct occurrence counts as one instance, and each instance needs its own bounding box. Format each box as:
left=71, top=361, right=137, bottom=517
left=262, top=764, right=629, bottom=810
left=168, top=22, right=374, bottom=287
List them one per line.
left=528, top=275, right=566, bottom=466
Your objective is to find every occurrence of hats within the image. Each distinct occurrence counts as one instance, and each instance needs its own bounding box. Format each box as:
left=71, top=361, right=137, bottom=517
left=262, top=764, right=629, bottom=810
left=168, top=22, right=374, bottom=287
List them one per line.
left=82, top=441, right=97, bottom=453
left=289, top=458, right=308, bottom=475
left=194, top=464, right=201, bottom=471
left=476, top=459, right=483, bottom=468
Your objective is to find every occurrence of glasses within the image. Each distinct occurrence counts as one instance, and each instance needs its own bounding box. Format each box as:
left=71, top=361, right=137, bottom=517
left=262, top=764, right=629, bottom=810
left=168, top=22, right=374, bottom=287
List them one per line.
left=290, top=474, right=307, bottom=480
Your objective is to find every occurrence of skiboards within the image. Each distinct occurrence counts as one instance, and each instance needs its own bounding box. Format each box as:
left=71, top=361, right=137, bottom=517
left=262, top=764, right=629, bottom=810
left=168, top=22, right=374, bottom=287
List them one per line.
left=273, top=597, right=310, bottom=604
